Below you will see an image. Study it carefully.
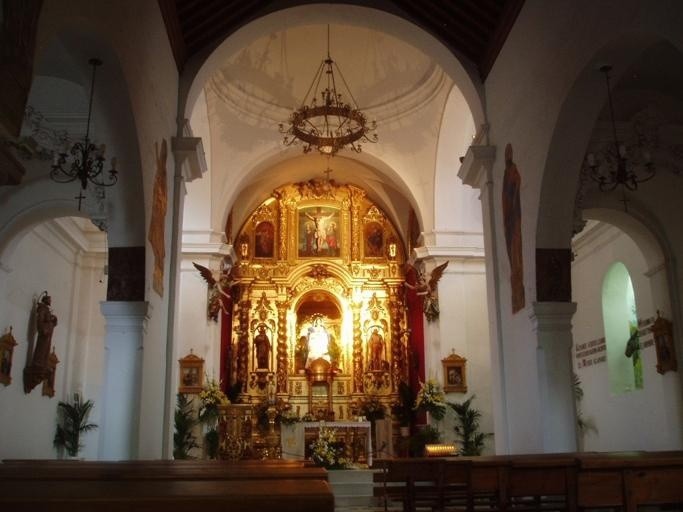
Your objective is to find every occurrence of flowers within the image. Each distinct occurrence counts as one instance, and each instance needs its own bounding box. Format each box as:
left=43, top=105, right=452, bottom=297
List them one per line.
left=309, top=419, right=347, bottom=469
left=199, top=373, right=231, bottom=425
left=411, top=367, right=446, bottom=420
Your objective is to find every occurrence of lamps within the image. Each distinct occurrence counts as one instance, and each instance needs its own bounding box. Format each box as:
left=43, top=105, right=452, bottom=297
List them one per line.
left=579, top=65, right=657, bottom=193
left=277, top=23, right=379, bottom=159
left=49, top=59, right=118, bottom=189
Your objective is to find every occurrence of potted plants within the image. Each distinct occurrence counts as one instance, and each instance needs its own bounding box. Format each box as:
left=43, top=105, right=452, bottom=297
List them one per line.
left=392, top=380, right=418, bottom=437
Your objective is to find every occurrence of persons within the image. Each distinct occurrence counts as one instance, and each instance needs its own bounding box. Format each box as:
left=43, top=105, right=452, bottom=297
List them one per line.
left=255, top=328, right=270, bottom=369
left=369, top=328, right=384, bottom=372
left=448, top=368, right=462, bottom=385
left=209, top=274, right=242, bottom=316
left=404, top=274, right=439, bottom=316
left=501, top=143, right=526, bottom=314
left=147, top=137, right=170, bottom=298
left=305, top=211, right=336, bottom=253
left=305, top=318, right=331, bottom=368
left=29, top=291, right=57, bottom=366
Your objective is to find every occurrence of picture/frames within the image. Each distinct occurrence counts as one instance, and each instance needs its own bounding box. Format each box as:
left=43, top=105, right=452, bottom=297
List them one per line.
left=178, top=349, right=205, bottom=394
left=441, top=348, right=468, bottom=394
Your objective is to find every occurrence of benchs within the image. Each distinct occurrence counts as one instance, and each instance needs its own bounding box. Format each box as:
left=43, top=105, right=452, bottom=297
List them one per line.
left=372, top=450, right=683, bottom=512
left=0, top=459, right=337, bottom=512
